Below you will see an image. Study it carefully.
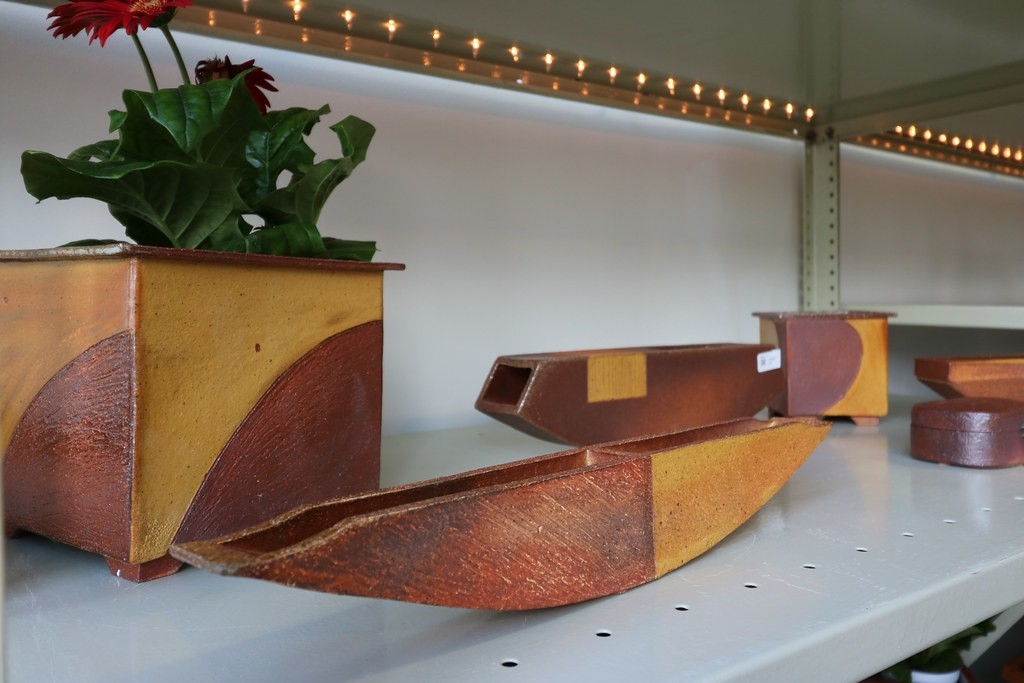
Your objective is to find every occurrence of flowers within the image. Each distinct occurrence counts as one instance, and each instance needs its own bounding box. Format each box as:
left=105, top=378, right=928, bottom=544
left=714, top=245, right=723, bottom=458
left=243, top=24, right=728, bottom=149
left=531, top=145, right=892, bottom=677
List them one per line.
left=20, top=1, right=378, bottom=261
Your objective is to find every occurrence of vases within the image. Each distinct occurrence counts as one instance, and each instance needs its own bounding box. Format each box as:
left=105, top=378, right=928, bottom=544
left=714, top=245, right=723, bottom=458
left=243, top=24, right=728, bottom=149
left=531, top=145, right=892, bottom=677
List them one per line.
left=0, top=242, right=407, bottom=585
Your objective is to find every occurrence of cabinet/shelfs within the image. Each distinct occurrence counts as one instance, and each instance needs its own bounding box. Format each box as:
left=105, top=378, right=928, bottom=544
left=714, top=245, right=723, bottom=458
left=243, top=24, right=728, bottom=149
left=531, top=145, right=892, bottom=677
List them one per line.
left=0, top=0, right=1024, bottom=683
